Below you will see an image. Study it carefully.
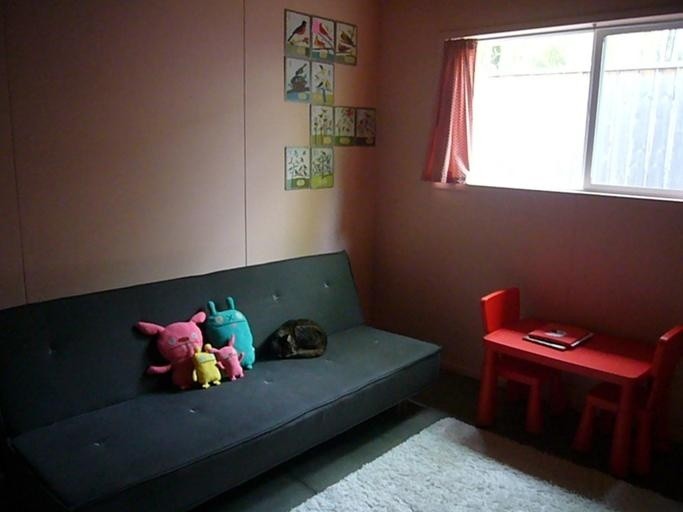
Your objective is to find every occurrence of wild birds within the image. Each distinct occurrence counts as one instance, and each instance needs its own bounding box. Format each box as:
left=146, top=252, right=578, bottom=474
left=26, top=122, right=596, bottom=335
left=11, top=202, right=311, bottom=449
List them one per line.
left=340, top=30, right=354, bottom=46
left=318, top=23, right=333, bottom=41
left=288, top=19, right=307, bottom=42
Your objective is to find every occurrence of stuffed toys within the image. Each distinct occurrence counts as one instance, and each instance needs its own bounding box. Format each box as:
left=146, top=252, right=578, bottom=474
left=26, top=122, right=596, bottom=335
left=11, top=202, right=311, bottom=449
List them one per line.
left=137, top=311, right=208, bottom=392
left=188, top=342, right=224, bottom=389
left=205, top=334, right=248, bottom=381
left=203, top=294, right=258, bottom=371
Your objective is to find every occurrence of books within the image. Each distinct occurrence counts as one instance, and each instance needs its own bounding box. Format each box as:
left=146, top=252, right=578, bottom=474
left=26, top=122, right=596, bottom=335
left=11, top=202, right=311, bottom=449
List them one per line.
left=527, top=322, right=596, bottom=348
left=523, top=331, right=567, bottom=350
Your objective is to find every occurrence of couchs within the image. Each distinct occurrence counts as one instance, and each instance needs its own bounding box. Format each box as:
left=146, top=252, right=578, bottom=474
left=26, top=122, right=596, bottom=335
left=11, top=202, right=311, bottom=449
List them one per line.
left=0, top=249, right=444, bottom=510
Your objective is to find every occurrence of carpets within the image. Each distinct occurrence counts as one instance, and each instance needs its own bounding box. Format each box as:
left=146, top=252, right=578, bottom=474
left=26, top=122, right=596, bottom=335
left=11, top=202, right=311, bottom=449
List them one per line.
left=285, top=413, right=682, bottom=511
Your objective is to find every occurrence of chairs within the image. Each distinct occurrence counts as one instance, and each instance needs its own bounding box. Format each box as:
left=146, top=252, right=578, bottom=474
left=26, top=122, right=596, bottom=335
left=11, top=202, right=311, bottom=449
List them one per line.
left=476, top=286, right=683, bottom=477
